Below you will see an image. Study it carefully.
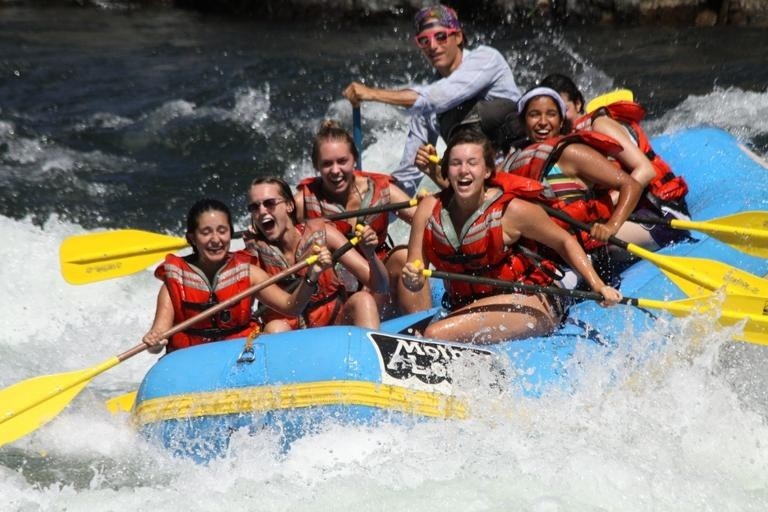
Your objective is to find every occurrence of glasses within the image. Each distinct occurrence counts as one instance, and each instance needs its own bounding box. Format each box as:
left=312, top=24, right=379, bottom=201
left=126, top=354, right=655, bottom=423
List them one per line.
left=248, top=198, right=286, bottom=212
left=414, top=28, right=456, bottom=48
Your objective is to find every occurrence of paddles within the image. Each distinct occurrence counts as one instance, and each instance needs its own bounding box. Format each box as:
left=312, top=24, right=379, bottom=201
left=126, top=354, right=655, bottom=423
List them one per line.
left=614, top=210, right=768, bottom=261
left=62, top=191, right=429, bottom=282
left=410, top=259, right=766, bottom=346
left=0, top=255, right=317, bottom=447
left=521, top=197, right=765, bottom=298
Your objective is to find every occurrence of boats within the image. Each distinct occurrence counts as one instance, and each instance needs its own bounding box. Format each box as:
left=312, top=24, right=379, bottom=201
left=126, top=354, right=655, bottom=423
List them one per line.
left=130, top=128, right=768, bottom=465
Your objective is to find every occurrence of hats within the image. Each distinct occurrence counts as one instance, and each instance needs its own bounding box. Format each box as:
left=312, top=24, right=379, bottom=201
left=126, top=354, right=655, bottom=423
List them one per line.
left=413, top=4, right=468, bottom=47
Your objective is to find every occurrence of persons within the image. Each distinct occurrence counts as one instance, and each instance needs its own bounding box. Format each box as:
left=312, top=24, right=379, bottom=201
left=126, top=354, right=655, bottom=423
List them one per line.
left=415, top=86, right=645, bottom=307
left=399, top=128, right=622, bottom=345
left=539, top=74, right=693, bottom=260
left=143, top=196, right=332, bottom=356
left=240, top=177, right=390, bottom=334
left=242, top=118, right=437, bottom=311
left=342, top=3, right=526, bottom=207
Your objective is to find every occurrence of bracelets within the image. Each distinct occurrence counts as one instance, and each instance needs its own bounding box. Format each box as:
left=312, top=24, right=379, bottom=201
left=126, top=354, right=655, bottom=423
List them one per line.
left=305, top=273, right=318, bottom=287
